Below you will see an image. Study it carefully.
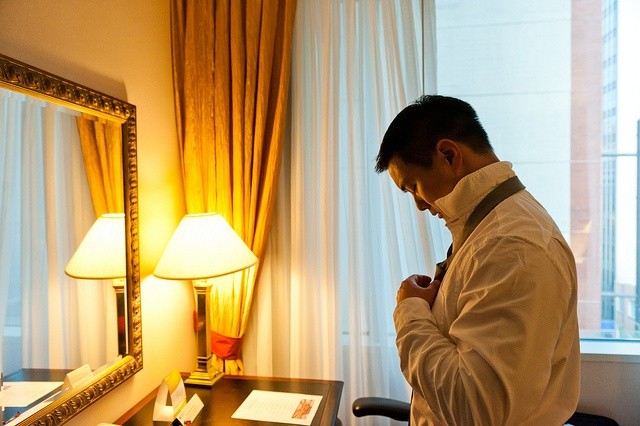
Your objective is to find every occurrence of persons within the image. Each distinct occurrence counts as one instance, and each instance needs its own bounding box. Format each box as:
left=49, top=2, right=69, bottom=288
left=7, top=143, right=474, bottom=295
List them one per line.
left=374, top=95, right=580, bottom=426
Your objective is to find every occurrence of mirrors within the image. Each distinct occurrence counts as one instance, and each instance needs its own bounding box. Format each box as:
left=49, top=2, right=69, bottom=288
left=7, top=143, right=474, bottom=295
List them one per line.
left=0, top=54, right=146, bottom=426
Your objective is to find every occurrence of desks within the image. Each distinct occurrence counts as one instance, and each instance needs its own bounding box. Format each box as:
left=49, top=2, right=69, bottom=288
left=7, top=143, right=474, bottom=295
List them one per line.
left=123, top=371, right=343, bottom=426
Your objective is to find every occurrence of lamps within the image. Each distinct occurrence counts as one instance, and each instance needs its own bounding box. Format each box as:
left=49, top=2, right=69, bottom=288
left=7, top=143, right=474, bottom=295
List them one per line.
left=154, top=213, right=258, bottom=383
left=65, top=209, right=127, bottom=358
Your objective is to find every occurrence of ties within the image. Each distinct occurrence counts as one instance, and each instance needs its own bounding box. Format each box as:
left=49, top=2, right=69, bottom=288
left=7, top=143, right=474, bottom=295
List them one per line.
left=434, top=175, right=526, bottom=282
